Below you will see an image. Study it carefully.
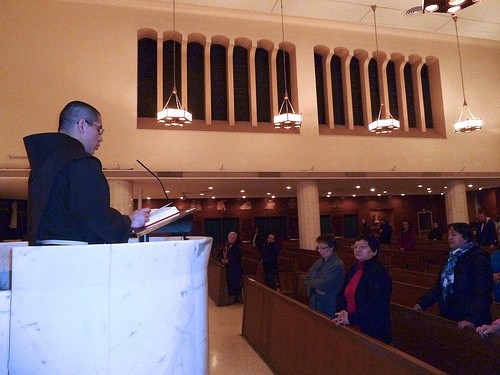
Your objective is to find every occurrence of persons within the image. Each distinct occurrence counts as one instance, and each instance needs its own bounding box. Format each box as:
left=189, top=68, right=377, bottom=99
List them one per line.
left=259, top=233, right=281, bottom=289
left=413, top=222, right=493, bottom=329
left=330, top=234, right=393, bottom=347
left=251, top=228, right=264, bottom=251
left=474, top=211, right=498, bottom=249
left=490, top=222, right=500, bottom=305
left=398, top=219, right=417, bottom=252
left=303, top=233, right=344, bottom=320
left=476, top=319, right=500, bottom=339
left=425, top=222, right=443, bottom=242
left=219, top=232, right=244, bottom=303
left=378, top=218, right=394, bottom=244
left=359, top=219, right=371, bottom=236
left=23, top=101, right=151, bottom=246
left=494, top=215, right=500, bottom=231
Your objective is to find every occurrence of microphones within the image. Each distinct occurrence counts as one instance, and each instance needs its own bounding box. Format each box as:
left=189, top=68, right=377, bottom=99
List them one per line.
left=136, top=159, right=171, bottom=207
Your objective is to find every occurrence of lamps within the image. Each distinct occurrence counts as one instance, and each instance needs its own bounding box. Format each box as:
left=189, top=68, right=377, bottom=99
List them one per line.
left=271, top=0, right=303, bottom=130
left=451, top=16, right=483, bottom=134
left=366, top=4, right=401, bottom=135
left=151, top=0, right=194, bottom=129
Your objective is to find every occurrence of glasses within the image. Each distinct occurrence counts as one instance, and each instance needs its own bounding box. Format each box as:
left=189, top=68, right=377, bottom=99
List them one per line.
left=352, top=244, right=368, bottom=249
left=76, top=118, right=105, bottom=136
left=316, top=245, right=330, bottom=250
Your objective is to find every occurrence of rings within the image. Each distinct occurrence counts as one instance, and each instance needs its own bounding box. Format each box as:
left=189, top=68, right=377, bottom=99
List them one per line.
left=340, top=320, right=343, bottom=324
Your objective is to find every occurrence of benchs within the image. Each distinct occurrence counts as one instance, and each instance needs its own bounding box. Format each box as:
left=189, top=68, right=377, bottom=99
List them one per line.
left=208, top=233, right=500, bottom=375
